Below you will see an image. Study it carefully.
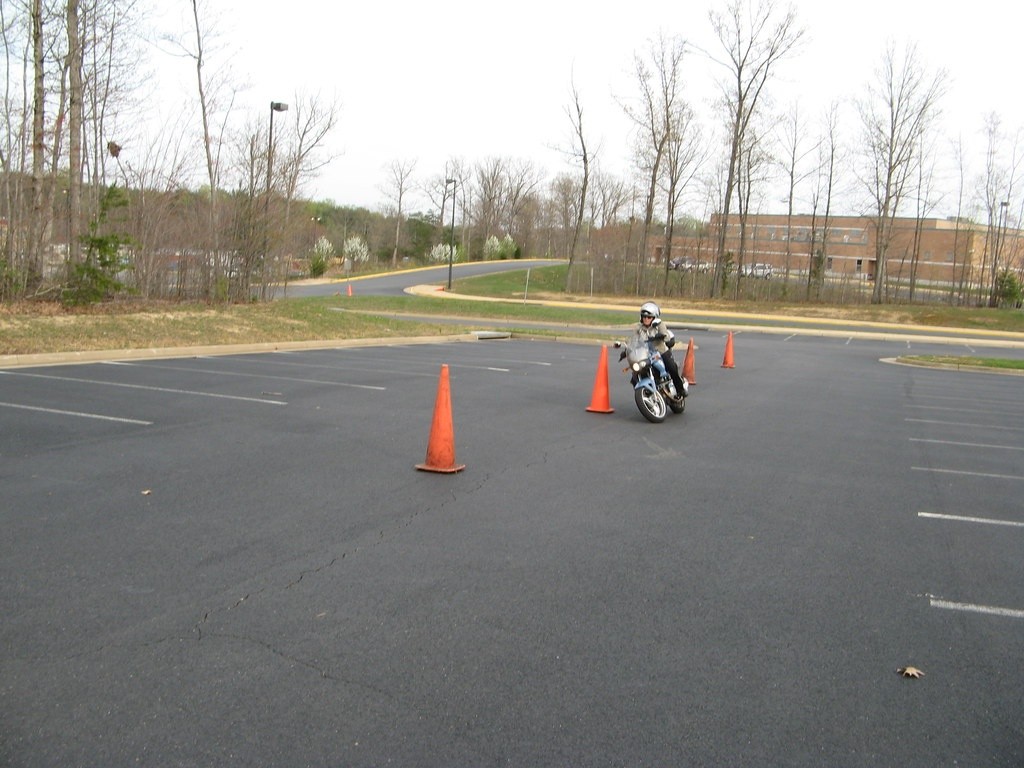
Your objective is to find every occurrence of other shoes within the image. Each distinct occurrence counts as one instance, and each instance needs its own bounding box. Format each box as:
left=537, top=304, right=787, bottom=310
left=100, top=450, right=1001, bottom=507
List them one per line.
left=681, top=389, right=689, bottom=396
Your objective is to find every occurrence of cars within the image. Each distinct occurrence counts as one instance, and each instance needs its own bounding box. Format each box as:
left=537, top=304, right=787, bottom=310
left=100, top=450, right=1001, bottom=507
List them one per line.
left=50, top=242, right=247, bottom=278
left=668, top=255, right=774, bottom=280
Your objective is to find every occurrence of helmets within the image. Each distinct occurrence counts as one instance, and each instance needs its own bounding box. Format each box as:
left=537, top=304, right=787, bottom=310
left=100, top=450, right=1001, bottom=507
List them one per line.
left=640, top=301, right=661, bottom=327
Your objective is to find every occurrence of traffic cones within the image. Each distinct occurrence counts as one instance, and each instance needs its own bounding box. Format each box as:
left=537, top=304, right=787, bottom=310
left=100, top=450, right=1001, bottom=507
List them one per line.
left=414, top=363, right=466, bottom=474
left=347, top=283, right=353, bottom=298
left=681, top=336, right=697, bottom=385
left=584, top=343, right=617, bottom=413
left=720, top=330, right=737, bottom=368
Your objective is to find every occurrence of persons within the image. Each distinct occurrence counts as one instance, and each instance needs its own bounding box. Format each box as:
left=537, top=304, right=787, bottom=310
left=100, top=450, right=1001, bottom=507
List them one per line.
left=620, top=302, right=686, bottom=396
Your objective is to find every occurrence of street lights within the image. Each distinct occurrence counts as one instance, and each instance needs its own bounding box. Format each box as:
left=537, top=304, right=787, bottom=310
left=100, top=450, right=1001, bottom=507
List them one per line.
left=310, top=215, right=321, bottom=248
left=988, top=202, right=1011, bottom=308
left=445, top=179, right=457, bottom=289
left=261, top=101, right=289, bottom=302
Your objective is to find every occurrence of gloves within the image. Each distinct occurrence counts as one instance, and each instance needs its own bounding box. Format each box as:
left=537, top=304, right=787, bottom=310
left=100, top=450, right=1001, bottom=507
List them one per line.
left=655, top=333, right=664, bottom=341
left=620, top=351, right=626, bottom=359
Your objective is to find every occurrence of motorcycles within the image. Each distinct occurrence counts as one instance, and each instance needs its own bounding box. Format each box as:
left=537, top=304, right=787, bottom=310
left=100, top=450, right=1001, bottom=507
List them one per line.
left=614, top=332, right=689, bottom=424
